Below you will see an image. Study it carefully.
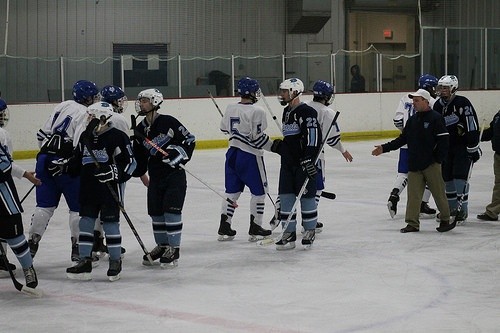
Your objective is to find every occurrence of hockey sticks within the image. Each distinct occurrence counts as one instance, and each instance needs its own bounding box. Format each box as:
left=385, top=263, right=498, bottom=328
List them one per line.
left=18, top=184, right=36, bottom=204
left=258, top=86, right=337, bottom=201
left=83, top=118, right=153, bottom=265
left=259, top=109, right=343, bottom=246
left=205, top=87, right=283, bottom=231
left=436, top=117, right=486, bottom=232
left=129, top=113, right=240, bottom=207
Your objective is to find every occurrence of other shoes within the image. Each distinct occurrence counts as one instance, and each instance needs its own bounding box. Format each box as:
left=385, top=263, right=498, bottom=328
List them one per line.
left=477, top=214, right=498, bottom=221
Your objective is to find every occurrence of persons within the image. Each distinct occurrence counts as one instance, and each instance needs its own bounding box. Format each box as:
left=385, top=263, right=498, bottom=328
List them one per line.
left=351, top=65, right=365, bottom=92
left=274, top=78, right=322, bottom=249
left=219, top=77, right=282, bottom=243
left=387, top=75, right=438, bottom=219
left=0, top=79, right=137, bottom=289
left=477, top=109, right=500, bottom=222
left=132, top=89, right=195, bottom=269
left=371, top=89, right=449, bottom=234
left=275, top=81, right=353, bottom=233
left=433, top=75, right=480, bottom=224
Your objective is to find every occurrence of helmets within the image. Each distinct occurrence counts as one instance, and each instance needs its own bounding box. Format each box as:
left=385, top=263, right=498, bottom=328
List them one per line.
left=438, top=75, right=459, bottom=99
left=0, top=98, right=10, bottom=127
left=279, top=78, right=304, bottom=105
left=313, top=80, right=334, bottom=105
left=86, top=101, right=113, bottom=123
left=72, top=80, right=98, bottom=105
left=238, top=77, right=261, bottom=102
left=418, top=74, right=438, bottom=97
left=100, top=85, right=128, bottom=113
left=136, top=89, right=163, bottom=116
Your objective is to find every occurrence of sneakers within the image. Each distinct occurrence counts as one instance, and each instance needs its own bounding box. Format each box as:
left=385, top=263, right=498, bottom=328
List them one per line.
left=0, top=255, right=17, bottom=277
left=66, top=230, right=125, bottom=281
left=23, top=264, right=38, bottom=289
left=27, top=233, right=41, bottom=260
left=160, top=246, right=180, bottom=268
left=143, top=243, right=169, bottom=265
left=386, top=188, right=467, bottom=233
left=217, top=208, right=323, bottom=250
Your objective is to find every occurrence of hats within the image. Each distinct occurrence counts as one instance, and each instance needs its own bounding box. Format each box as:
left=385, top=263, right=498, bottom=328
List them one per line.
left=408, top=89, right=430, bottom=104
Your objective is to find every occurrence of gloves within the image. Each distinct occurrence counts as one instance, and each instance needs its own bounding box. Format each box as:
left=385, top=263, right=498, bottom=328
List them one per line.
left=300, top=156, right=317, bottom=182
left=94, top=164, right=119, bottom=183
left=466, top=144, right=482, bottom=163
left=162, top=144, right=189, bottom=168
left=271, top=139, right=283, bottom=155
left=49, top=158, right=71, bottom=176
